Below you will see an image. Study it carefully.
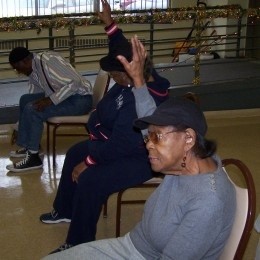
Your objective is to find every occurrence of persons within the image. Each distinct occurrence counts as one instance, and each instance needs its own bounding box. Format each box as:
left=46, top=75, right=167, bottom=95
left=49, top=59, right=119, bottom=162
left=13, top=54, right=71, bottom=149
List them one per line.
left=39, top=1, right=168, bottom=253
left=6, top=47, right=93, bottom=172
left=41, top=35, right=235, bottom=260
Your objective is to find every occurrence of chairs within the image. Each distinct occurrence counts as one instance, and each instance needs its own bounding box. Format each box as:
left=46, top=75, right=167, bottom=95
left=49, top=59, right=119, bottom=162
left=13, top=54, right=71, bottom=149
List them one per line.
left=45, top=67, right=256, bottom=260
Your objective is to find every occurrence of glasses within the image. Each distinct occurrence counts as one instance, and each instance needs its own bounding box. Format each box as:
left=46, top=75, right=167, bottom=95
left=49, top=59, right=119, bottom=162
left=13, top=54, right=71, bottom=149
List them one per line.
left=144, top=129, right=186, bottom=145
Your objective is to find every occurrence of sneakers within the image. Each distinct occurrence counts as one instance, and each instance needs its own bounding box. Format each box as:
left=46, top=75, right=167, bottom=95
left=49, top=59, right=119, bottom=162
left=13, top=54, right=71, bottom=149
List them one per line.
left=40, top=209, right=71, bottom=223
left=48, top=243, right=72, bottom=254
left=7, top=150, right=43, bottom=172
left=9, top=147, right=44, bottom=156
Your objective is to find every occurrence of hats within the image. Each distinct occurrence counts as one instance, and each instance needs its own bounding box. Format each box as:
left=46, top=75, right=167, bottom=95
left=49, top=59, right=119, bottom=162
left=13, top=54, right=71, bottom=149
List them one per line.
left=142, top=97, right=207, bottom=137
left=100, top=45, right=135, bottom=73
